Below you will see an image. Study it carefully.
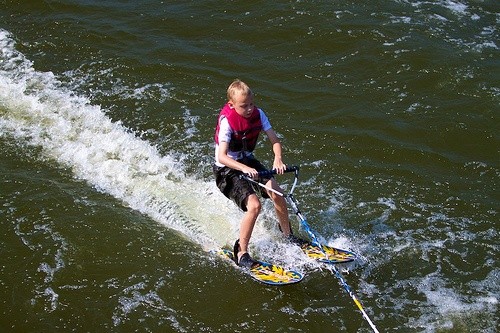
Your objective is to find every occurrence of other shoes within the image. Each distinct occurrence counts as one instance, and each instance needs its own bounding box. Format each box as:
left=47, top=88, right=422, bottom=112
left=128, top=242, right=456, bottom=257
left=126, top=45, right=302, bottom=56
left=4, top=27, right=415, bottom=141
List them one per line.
left=234, top=252, right=264, bottom=270
left=280, top=233, right=311, bottom=250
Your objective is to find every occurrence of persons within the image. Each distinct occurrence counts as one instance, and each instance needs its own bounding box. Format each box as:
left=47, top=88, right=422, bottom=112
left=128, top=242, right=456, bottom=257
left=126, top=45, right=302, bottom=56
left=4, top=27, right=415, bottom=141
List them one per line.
left=214, top=79, right=310, bottom=270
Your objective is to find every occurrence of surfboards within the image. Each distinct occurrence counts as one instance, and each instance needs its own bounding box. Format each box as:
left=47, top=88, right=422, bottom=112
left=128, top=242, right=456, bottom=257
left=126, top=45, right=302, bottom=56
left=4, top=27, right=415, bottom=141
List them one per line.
left=271, top=232, right=357, bottom=263
left=205, top=246, right=303, bottom=286
